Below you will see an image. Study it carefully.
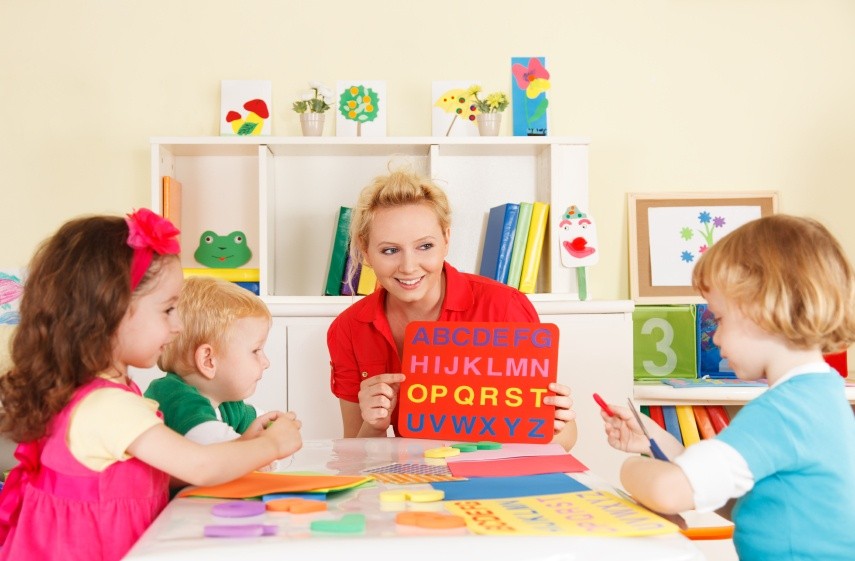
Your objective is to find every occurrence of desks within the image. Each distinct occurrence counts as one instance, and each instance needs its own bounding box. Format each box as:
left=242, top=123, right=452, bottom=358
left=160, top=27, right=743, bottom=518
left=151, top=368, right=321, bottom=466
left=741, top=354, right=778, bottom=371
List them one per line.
left=116, top=434, right=743, bottom=561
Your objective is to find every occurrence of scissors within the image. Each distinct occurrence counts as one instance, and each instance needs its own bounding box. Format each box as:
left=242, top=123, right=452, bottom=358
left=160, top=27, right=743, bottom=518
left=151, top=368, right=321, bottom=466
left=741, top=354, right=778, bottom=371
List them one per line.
left=628, top=399, right=668, bottom=462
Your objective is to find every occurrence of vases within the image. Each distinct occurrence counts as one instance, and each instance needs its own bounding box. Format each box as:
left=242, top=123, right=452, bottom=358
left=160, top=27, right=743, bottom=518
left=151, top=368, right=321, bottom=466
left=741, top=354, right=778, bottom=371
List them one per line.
left=475, top=113, right=503, bottom=136
left=298, top=113, right=326, bottom=138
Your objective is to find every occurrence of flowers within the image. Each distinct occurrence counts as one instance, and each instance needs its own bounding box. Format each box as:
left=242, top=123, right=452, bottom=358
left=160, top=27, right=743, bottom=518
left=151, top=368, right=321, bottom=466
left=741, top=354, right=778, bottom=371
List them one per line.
left=290, top=79, right=336, bottom=112
left=468, top=83, right=510, bottom=112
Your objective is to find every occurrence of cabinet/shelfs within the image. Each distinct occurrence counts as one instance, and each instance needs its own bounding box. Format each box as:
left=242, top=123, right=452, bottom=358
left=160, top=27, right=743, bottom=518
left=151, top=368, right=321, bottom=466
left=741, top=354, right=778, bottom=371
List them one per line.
left=150, top=127, right=641, bottom=475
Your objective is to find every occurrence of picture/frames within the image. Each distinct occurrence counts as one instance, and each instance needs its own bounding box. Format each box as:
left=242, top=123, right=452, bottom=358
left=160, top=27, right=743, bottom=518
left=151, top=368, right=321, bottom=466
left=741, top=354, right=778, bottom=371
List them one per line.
left=629, top=187, right=782, bottom=306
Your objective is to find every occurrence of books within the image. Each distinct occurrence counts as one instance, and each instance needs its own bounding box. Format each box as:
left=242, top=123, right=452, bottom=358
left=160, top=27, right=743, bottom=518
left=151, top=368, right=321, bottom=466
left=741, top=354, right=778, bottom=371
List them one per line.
left=326, top=200, right=547, bottom=297
left=639, top=405, right=731, bottom=461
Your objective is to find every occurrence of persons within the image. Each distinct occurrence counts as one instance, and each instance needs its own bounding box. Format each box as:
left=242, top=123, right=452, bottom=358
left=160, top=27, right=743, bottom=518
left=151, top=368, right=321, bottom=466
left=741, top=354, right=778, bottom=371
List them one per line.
left=0, top=207, right=302, bottom=561
left=601, top=213, right=855, bottom=561
left=328, top=176, right=576, bottom=440
